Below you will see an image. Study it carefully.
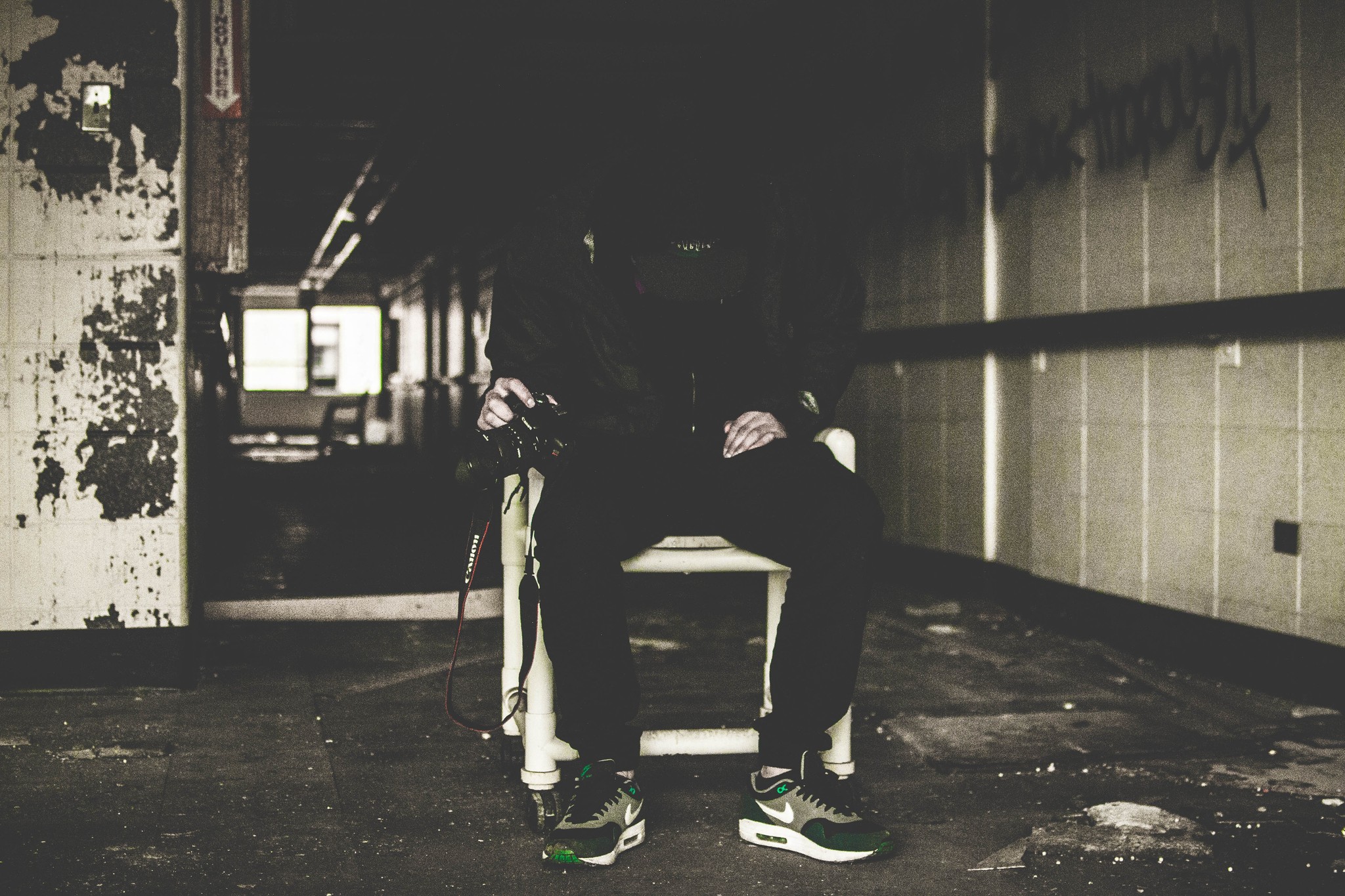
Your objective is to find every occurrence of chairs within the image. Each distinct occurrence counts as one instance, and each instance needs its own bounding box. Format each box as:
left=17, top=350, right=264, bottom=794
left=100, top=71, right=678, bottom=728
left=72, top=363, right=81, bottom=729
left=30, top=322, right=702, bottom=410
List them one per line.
left=491, top=396, right=867, bottom=836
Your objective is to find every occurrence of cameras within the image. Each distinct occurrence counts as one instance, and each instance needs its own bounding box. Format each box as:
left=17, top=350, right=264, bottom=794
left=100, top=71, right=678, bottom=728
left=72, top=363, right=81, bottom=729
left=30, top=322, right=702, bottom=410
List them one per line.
left=466, top=391, right=570, bottom=481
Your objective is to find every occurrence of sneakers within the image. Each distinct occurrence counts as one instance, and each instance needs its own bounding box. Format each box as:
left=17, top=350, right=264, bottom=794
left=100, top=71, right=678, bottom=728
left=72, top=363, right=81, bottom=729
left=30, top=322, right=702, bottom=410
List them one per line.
left=542, top=759, right=647, bottom=867
left=739, top=750, right=899, bottom=863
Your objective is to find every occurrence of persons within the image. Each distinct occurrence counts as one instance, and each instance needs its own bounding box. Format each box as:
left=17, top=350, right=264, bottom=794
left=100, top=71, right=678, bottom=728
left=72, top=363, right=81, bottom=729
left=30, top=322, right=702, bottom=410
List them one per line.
left=476, top=146, right=893, bottom=866
